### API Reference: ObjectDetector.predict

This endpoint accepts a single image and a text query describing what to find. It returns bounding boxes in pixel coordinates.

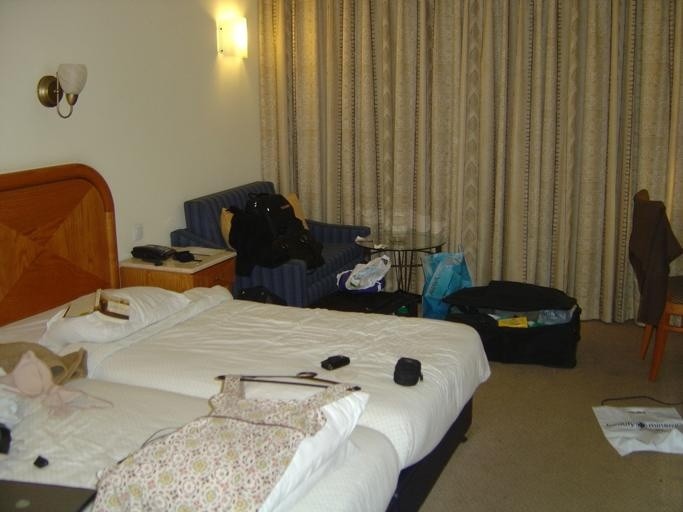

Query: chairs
[171,181,371,308]
[633,189,683,383]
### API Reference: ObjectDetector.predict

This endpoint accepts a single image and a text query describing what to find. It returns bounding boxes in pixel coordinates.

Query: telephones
[131,244,177,260]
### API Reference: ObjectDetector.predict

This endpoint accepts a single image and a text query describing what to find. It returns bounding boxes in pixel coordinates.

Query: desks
[354,229,446,312]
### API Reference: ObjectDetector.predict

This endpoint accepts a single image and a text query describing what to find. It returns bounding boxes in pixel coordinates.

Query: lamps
[217,5,250,62]
[38,64,85,118]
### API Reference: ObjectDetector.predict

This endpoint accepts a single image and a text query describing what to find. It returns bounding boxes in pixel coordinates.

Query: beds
[0,381,398,512]
[0,162,491,503]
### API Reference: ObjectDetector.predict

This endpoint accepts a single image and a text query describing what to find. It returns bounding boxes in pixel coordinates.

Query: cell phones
[321,356,350,369]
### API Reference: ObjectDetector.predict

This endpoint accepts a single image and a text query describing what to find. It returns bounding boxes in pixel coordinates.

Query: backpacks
[246,191,305,266]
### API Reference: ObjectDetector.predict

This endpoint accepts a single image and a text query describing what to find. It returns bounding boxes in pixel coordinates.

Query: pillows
[220,193,310,249]
[47,286,187,335]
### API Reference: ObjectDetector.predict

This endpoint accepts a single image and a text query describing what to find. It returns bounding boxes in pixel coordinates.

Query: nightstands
[120,249,238,300]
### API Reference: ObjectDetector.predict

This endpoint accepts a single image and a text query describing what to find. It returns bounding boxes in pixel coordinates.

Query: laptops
[0,479,96,512]
